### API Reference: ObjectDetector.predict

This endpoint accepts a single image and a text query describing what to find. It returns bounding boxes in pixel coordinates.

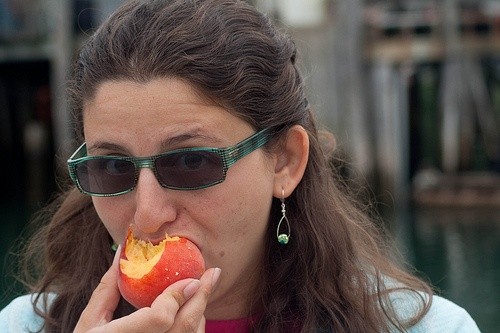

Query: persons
[0,0,482,333]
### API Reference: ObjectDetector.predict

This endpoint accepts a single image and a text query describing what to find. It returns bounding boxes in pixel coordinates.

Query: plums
[118,228,205,310]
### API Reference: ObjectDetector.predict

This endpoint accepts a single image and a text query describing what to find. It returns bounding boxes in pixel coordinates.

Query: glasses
[67,127,277,198]
